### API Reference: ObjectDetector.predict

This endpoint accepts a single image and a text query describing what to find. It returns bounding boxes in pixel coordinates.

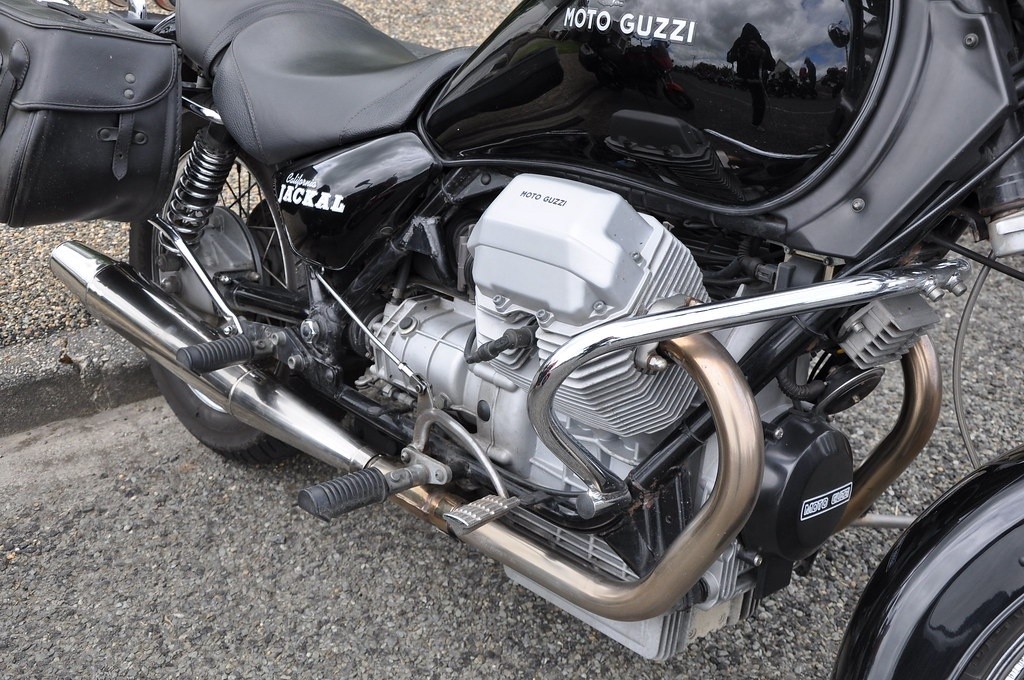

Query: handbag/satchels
[0,0,181,228]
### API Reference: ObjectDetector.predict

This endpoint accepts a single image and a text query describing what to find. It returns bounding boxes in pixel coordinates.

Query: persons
[726,23,816,132]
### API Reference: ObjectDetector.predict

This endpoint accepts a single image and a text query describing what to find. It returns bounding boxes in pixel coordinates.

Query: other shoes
[155,0,175,11]
[108,0,129,7]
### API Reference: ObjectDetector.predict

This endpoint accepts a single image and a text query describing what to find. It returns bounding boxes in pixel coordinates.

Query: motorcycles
[47,2,1024,679]
